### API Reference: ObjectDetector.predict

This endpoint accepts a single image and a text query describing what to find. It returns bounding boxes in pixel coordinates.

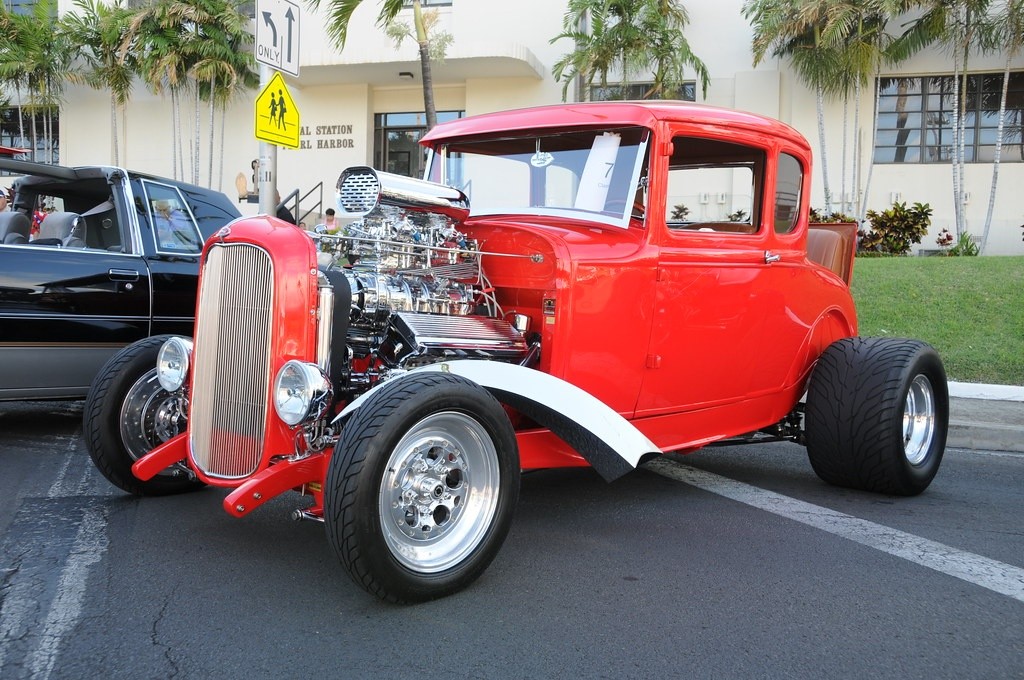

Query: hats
[0,186,10,196]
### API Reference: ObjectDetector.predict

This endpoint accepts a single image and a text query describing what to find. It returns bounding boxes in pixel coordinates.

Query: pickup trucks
[0,156,245,405]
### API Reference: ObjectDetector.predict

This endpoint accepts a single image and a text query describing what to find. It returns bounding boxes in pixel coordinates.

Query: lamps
[399,72,414,79]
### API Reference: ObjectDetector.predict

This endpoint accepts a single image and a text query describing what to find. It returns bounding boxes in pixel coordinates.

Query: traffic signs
[253,0,301,79]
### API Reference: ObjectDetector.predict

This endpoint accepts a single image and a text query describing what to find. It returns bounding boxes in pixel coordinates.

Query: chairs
[37,212,89,248]
[806,229,844,278]
[677,221,755,234]
[0,212,31,244]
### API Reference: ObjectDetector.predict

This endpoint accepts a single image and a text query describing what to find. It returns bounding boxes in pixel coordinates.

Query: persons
[154,199,195,245]
[276,188,343,230]
[0,190,11,212]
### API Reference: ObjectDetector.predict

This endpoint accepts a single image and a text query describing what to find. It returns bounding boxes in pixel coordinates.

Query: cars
[79,97,953,608]
